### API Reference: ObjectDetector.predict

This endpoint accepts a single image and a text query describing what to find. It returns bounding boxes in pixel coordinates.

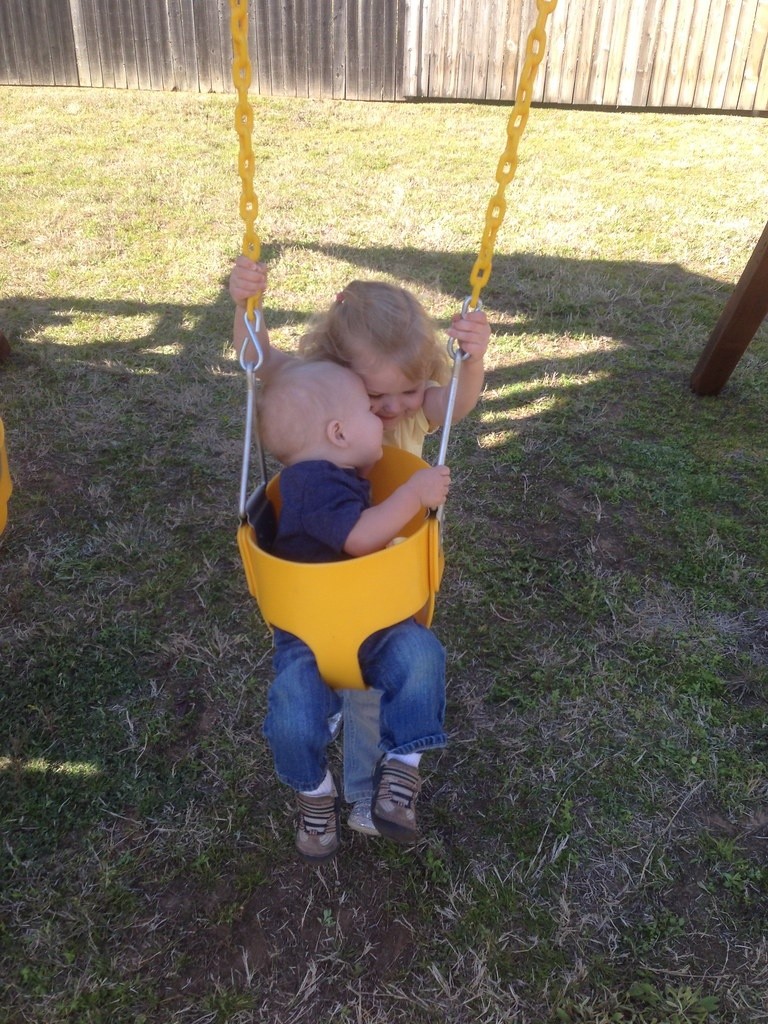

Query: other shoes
[346,813,379,835]
[326,710,342,743]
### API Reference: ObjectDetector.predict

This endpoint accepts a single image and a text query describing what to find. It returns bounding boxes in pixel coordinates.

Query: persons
[228,253,492,838]
[259,357,452,860]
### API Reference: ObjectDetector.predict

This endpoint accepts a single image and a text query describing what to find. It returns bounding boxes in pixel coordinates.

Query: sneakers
[372,757,422,831]
[294,790,338,857]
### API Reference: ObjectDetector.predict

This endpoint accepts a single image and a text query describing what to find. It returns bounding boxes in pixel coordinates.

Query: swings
[227,1,558,694]
